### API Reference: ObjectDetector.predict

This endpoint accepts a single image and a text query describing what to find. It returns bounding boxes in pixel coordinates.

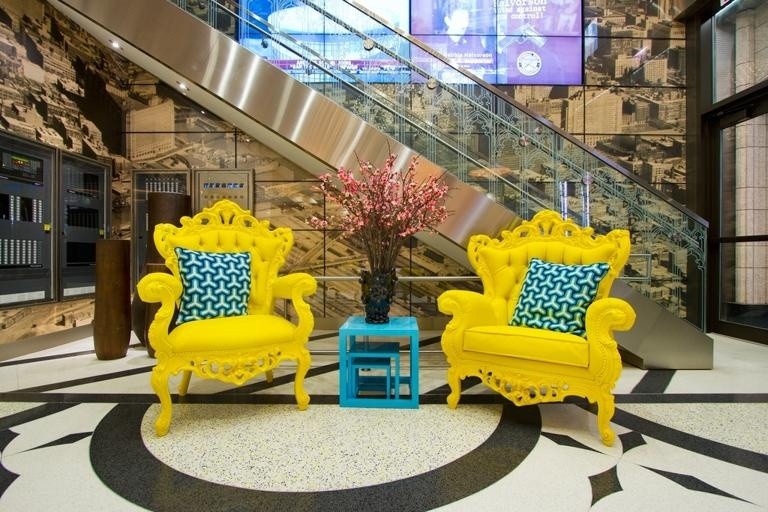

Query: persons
[433,1,495,85]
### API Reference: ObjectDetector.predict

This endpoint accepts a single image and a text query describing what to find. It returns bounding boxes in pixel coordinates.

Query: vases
[361,269,398,324]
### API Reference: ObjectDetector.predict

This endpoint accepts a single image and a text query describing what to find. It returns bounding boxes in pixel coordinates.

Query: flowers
[299,140,457,273]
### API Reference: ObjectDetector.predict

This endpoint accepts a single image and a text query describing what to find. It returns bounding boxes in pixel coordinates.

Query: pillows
[174,247,252,326]
[510,256,610,339]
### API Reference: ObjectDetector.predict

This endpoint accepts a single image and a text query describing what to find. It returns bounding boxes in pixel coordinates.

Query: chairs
[437,210,631,447]
[137,200,319,435]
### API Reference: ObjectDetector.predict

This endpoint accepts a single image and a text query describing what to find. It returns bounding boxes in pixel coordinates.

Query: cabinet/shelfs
[59,148,113,302]
[0,131,58,312]
